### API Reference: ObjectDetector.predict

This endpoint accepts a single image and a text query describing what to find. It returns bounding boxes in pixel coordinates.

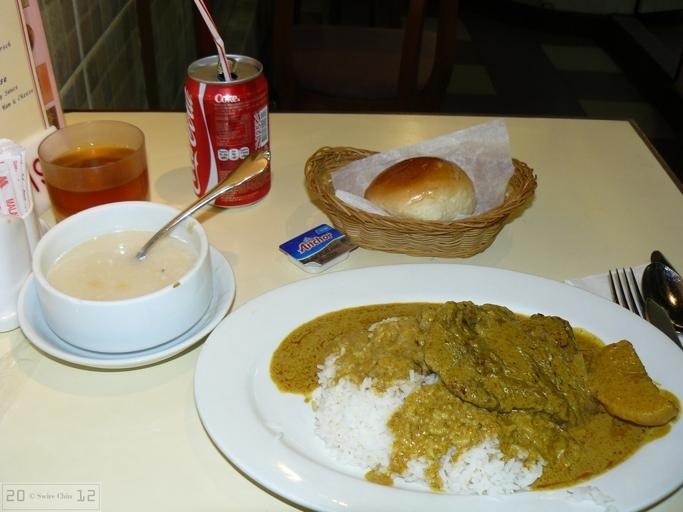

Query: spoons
[641,263,683,329]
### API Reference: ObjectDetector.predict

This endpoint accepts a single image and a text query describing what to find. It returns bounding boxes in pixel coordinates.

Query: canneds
[183,54,273,208]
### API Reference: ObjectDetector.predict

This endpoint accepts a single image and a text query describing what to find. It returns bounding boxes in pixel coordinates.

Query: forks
[608,267,646,320]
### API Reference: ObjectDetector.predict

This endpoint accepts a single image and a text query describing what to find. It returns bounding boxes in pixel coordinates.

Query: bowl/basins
[32,197,213,355]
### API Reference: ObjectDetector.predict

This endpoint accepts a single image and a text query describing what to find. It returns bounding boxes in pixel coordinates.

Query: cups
[37,120,149,223]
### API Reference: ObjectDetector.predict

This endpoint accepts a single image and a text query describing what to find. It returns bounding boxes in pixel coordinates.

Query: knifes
[651,251,681,283]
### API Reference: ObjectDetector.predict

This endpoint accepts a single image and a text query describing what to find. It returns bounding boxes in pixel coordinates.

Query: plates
[17,236,236,371]
[195,265,683,512]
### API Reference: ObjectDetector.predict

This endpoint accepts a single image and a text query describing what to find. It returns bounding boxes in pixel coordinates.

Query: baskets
[305,147,538,258]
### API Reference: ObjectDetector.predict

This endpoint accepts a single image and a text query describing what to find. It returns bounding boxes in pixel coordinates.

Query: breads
[362,157,478,223]
